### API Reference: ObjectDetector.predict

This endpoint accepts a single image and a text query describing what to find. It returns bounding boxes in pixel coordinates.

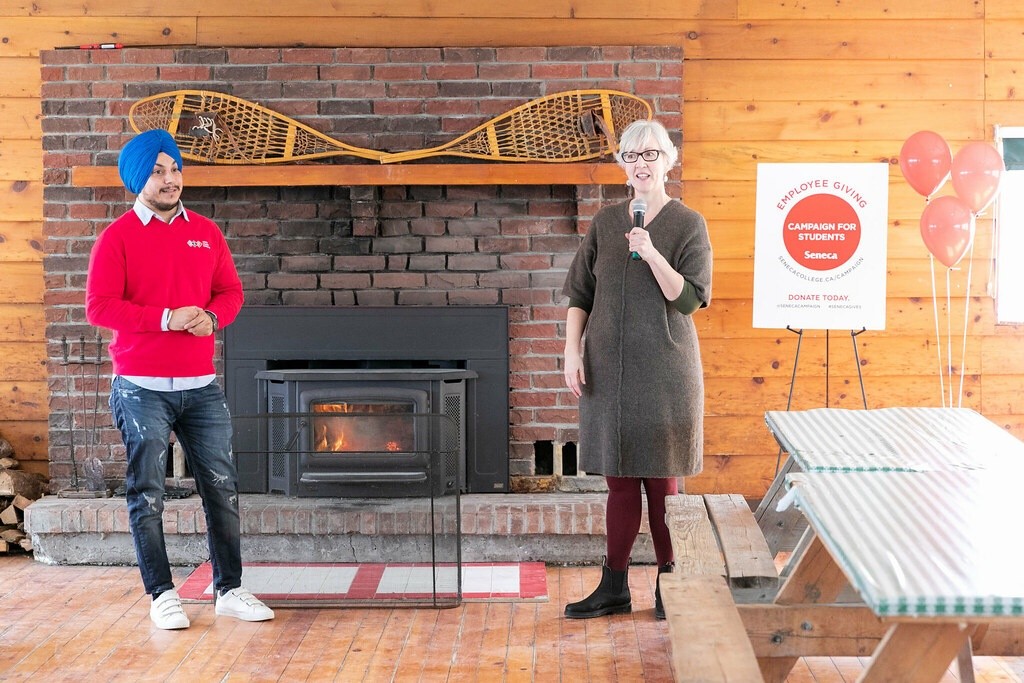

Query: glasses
[621,148,663,164]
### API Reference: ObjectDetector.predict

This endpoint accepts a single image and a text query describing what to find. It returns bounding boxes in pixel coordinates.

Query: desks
[783,467,1023,625]
[764,404,1023,472]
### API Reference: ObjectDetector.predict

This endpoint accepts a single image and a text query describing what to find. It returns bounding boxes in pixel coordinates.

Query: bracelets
[165,308,174,326]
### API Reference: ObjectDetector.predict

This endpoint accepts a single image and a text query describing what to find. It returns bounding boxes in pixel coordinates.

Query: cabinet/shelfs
[68,164,631,188]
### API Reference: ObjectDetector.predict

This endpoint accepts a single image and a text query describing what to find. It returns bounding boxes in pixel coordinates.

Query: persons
[562,120,714,619]
[84,131,277,630]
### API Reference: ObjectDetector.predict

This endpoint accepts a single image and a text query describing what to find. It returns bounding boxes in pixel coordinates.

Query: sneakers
[213,588,275,624]
[149,590,191,632]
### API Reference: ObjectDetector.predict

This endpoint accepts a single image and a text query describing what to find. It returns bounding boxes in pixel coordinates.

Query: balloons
[899,130,953,198]
[920,195,976,269]
[951,143,1005,218]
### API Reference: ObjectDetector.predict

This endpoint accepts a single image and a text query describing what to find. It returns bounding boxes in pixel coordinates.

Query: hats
[117,129,182,196]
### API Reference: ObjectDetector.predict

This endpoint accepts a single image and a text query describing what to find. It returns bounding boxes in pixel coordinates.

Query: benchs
[663,491,781,588]
[658,572,768,683]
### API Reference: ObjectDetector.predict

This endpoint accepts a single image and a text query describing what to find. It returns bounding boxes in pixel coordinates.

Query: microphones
[631,198,648,261]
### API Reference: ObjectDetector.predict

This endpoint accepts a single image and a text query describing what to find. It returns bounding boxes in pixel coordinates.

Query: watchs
[205,311,218,333]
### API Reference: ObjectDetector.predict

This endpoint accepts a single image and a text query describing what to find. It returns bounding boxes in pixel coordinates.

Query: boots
[564,556,633,619]
[655,563,675,618]
